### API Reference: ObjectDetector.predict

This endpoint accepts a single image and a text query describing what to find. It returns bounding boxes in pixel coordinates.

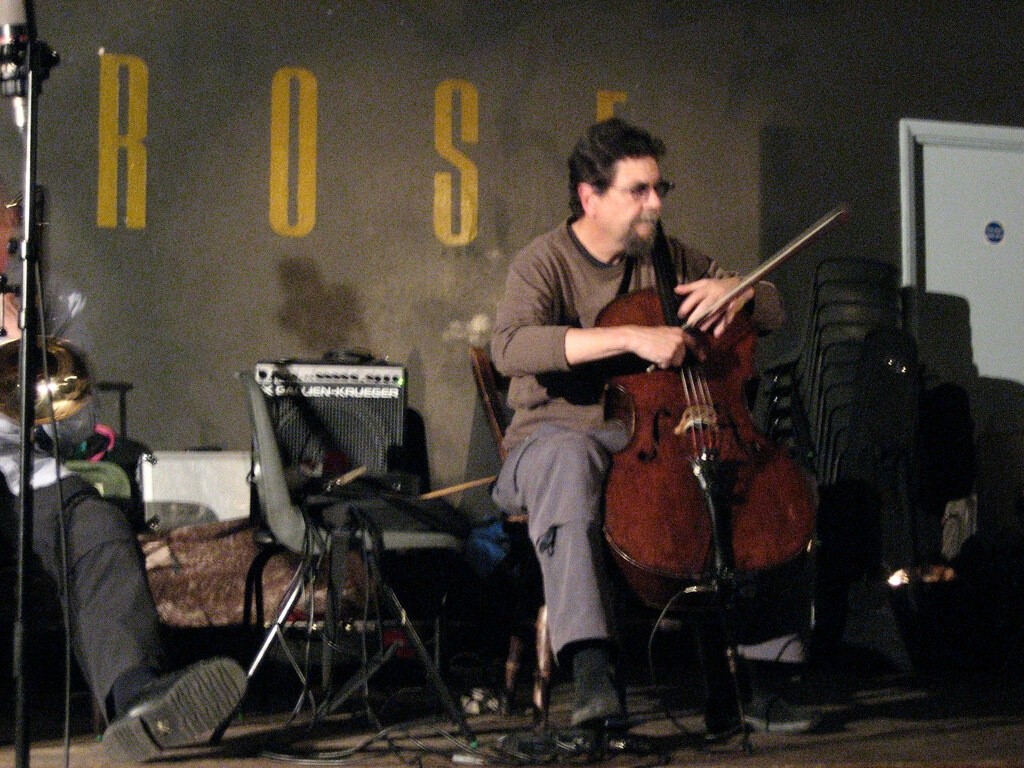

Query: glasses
[611,180,677,203]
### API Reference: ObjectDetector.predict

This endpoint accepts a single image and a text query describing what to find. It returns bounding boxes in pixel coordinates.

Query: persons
[490,120,815,735]
[0,194,247,764]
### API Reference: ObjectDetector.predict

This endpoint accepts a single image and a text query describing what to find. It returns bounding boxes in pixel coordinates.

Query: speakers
[248,361,407,534]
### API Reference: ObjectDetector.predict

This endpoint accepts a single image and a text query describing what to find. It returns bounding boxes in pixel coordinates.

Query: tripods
[211,502,482,752]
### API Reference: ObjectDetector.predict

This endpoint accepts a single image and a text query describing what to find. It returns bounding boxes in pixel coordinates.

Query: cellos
[572,174,832,761]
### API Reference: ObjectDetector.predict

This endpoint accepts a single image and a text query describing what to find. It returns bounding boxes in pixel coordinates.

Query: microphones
[0,0,32,132]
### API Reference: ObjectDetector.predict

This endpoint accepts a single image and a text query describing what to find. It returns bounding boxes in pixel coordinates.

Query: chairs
[469,346,727,731]
[765,255,930,647]
[242,372,462,711]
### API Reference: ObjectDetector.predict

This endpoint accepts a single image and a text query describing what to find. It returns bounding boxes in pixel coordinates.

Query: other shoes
[570,648,629,728]
[101,658,247,765]
[730,677,811,734]
